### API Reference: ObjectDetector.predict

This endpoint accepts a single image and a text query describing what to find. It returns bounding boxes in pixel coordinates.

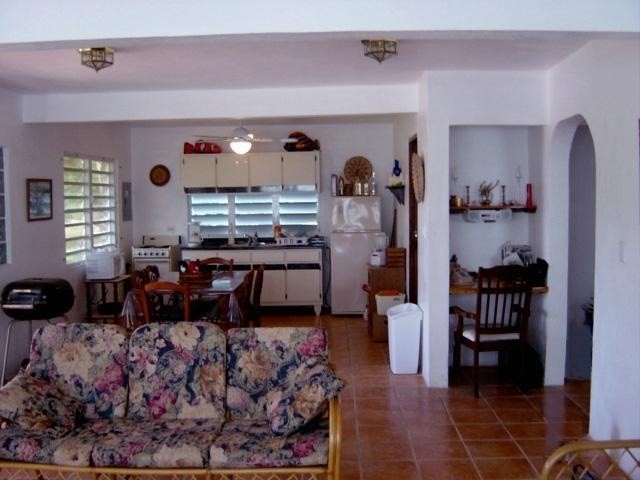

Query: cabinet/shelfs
[252,249,323,305]
[181,154,249,188]
[367,266,405,343]
[248,150,321,187]
[181,249,251,304]
[450,206,548,295]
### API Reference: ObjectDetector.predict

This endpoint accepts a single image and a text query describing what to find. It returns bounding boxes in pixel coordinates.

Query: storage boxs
[375,291,406,316]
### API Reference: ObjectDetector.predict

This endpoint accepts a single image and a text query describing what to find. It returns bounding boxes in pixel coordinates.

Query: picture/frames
[26,179,53,221]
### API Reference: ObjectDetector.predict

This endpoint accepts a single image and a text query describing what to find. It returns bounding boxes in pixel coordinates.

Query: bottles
[272,222,282,242]
[228,223,236,245]
[328,171,379,197]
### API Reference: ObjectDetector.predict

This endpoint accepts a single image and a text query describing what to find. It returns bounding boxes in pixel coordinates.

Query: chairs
[449,265,531,398]
[538,440,640,480]
[130,257,264,329]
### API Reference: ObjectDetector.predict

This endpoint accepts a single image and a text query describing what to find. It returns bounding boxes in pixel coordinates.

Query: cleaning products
[275,220,282,240]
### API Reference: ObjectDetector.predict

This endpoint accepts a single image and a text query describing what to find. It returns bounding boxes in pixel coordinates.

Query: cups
[186,221,205,249]
[188,259,201,273]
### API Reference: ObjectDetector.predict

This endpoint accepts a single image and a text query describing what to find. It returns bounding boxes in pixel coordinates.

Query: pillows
[0,368,83,438]
[265,355,344,438]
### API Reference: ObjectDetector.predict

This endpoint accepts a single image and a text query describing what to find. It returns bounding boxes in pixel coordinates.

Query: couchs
[0,321,341,480]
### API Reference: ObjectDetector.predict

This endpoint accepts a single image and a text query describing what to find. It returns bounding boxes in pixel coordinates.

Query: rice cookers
[369,247,385,268]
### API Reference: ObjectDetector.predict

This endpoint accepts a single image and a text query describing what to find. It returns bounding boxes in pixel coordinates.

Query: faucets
[243,234,257,243]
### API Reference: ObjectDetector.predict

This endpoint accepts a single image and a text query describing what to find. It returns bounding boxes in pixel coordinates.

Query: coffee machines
[328,197,389,317]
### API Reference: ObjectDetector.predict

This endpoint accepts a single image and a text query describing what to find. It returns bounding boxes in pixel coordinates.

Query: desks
[1,315,71,388]
[81,274,130,324]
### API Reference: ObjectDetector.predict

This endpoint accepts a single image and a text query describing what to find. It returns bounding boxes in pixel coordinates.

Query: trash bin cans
[387,302,422,375]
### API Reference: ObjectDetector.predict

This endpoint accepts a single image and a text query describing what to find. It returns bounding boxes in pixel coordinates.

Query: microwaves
[83,252,128,280]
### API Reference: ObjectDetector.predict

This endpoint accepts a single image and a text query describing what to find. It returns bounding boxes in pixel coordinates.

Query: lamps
[79,47,115,72]
[361,39,400,63]
[230,142,251,154]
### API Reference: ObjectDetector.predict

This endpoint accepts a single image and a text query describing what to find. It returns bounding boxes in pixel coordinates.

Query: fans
[192,121,297,145]
